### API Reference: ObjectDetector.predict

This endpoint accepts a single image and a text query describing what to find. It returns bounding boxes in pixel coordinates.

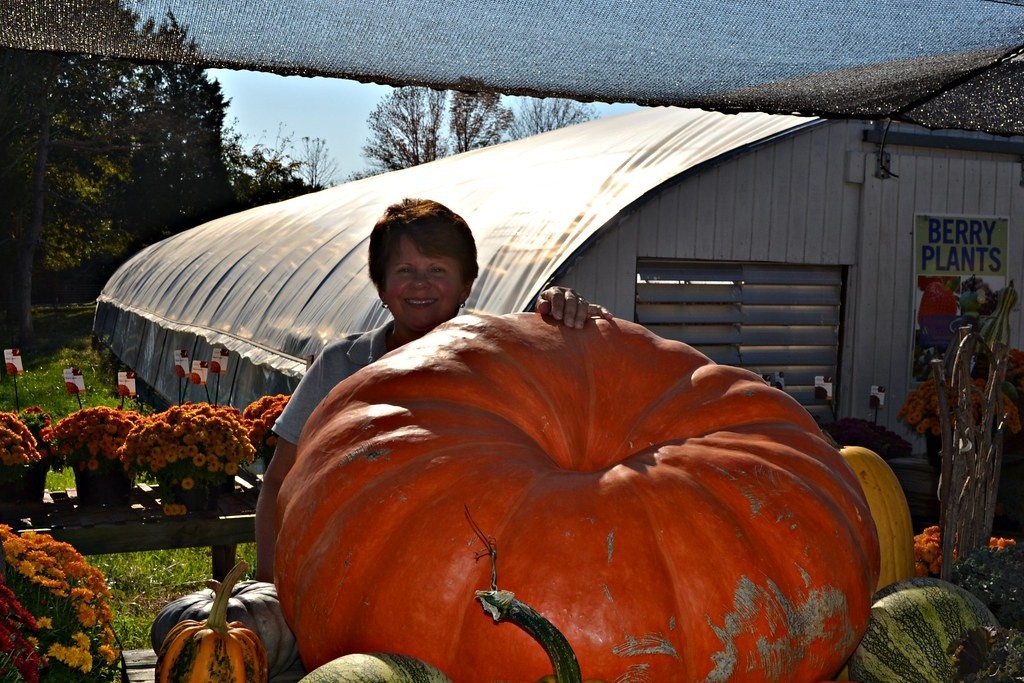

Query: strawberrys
[918,276,961,328]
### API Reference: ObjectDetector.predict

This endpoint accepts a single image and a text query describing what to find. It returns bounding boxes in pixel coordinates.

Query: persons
[250,197,617,586]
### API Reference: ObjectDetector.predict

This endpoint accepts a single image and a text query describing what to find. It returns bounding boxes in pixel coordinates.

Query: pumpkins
[973,278,1020,354]
[272,308,879,682]
[820,429,914,593]
[295,651,455,682]
[849,577,1004,682]
[149,561,304,683]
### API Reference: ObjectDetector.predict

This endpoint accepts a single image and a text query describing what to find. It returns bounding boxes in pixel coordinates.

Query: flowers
[49,405,146,473]
[241,393,292,459]
[0,405,54,483]
[116,400,257,516]
[0,523,123,683]
[910,524,1017,579]
[895,373,1022,435]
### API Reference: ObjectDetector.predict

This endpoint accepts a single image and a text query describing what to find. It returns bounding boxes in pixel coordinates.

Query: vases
[154,457,236,510]
[924,429,956,469]
[0,460,49,503]
[70,460,135,510]
